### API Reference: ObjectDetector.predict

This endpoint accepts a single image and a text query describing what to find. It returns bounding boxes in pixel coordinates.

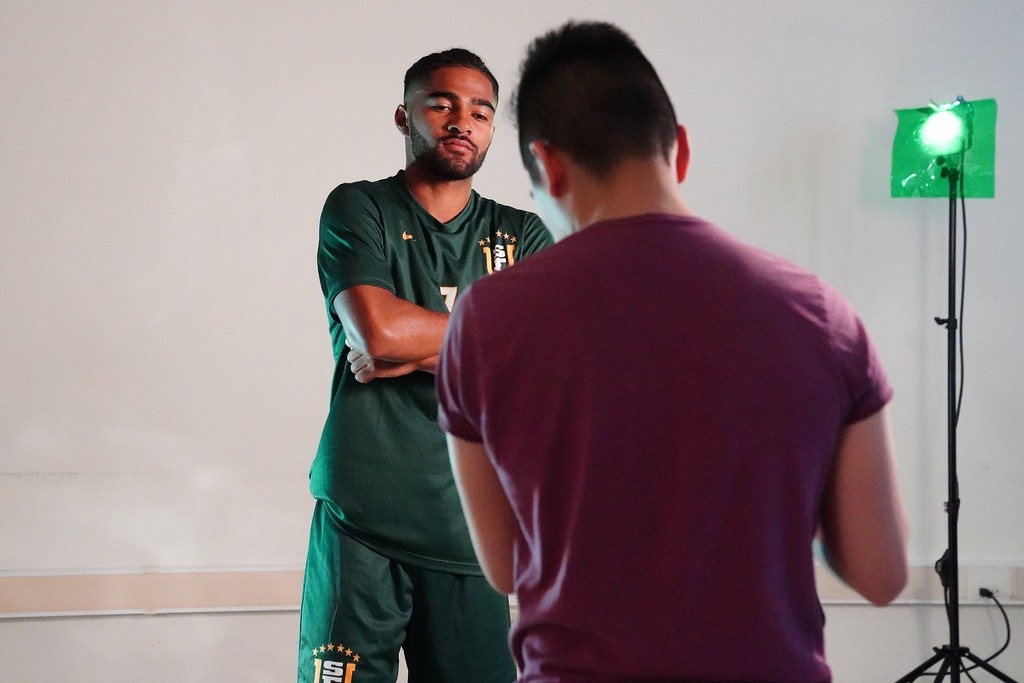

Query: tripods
[898,172,1013,683]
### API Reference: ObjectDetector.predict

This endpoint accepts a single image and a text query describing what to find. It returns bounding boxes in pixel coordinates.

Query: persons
[296,47,554,683]
[436,19,906,683]
